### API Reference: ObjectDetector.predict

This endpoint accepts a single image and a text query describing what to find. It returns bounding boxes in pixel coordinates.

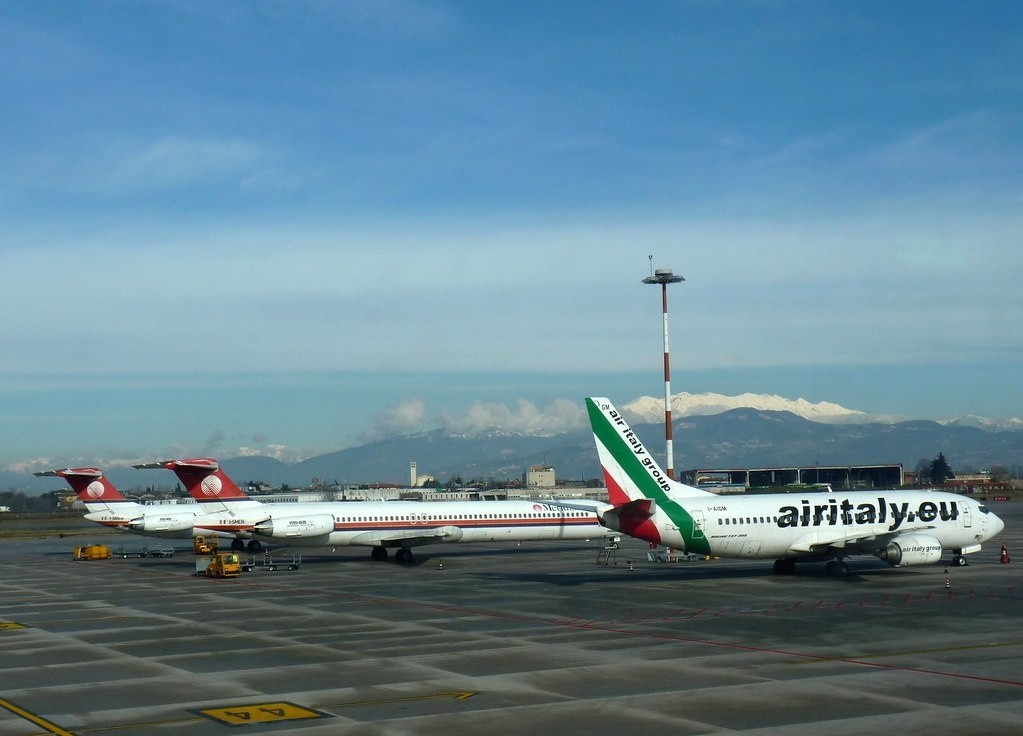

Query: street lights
[640,253,686,563]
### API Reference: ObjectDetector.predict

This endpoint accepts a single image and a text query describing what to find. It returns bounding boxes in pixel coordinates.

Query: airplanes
[32,456,626,564]
[529,396,1005,577]
